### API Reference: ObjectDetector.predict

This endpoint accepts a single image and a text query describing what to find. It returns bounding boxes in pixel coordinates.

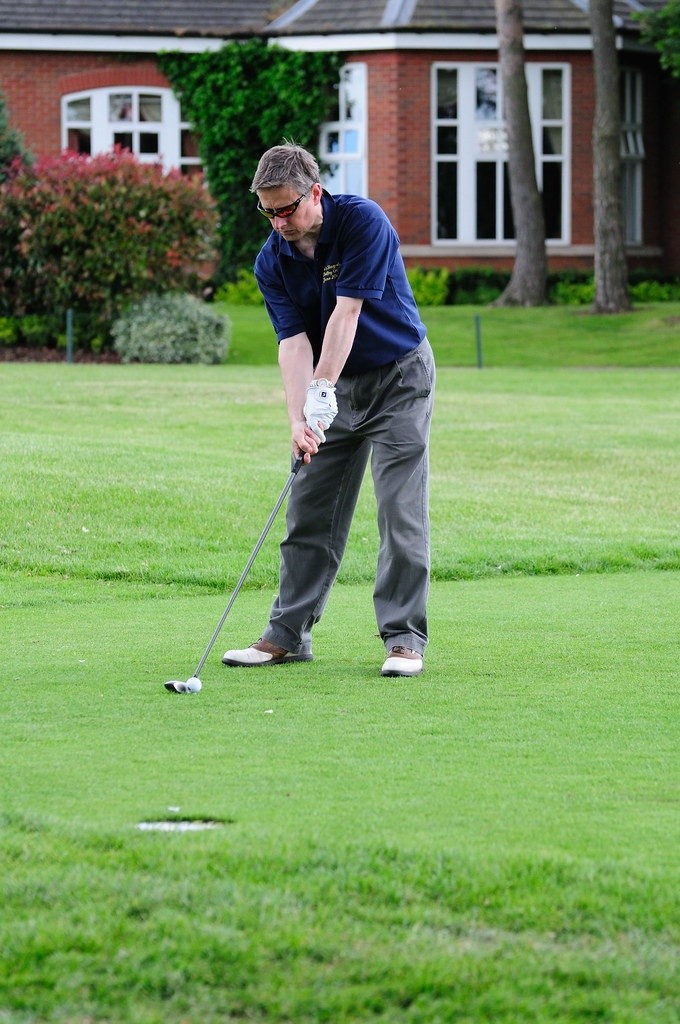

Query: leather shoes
[222,639,313,667]
[382,646,423,676]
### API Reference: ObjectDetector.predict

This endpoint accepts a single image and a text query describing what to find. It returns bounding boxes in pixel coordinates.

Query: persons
[219,136,433,677]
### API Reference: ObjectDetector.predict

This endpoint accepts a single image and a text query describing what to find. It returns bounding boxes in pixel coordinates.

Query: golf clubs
[163,427,312,695]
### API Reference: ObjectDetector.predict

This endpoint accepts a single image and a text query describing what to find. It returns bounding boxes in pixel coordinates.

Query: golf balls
[184,677,202,694]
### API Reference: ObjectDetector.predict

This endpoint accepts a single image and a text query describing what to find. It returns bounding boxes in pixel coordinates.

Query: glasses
[257,195,305,219]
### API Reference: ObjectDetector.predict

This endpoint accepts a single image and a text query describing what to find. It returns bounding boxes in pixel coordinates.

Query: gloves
[303,386,338,444]
